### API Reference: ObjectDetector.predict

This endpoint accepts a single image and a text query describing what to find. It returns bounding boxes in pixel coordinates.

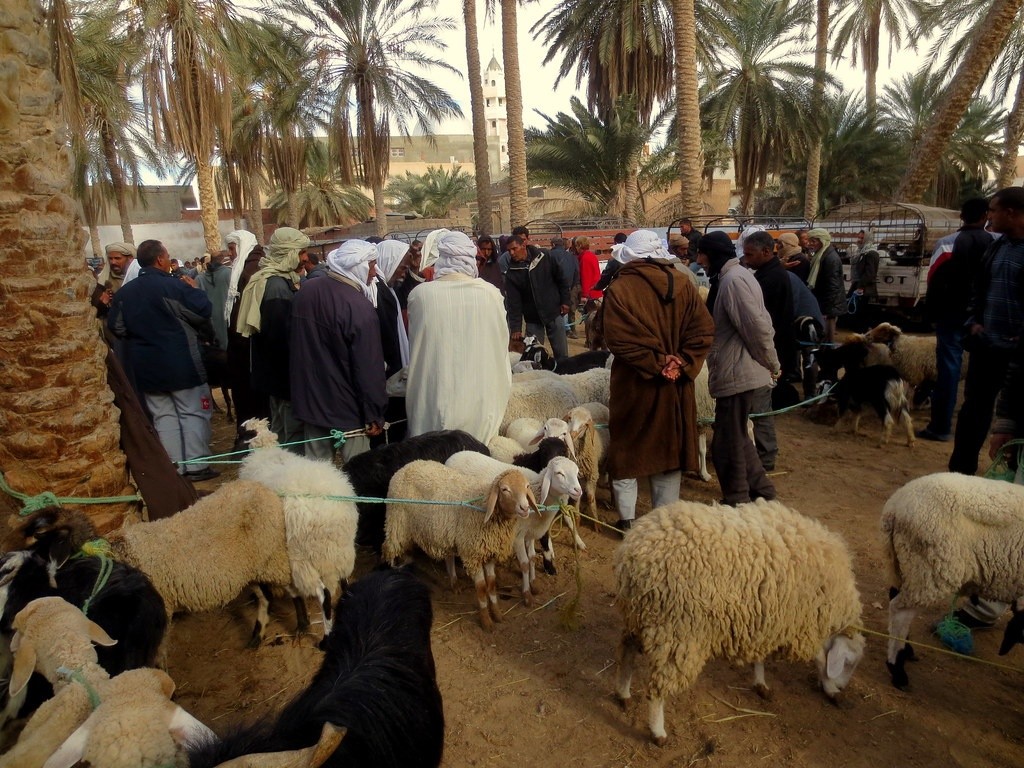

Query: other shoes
[917,429,950,443]
[187,466,220,481]
[933,605,994,634]
[616,517,634,541]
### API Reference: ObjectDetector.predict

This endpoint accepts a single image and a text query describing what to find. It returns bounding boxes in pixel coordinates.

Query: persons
[695,233,784,507]
[91,226,631,548]
[602,231,717,539]
[668,187,1024,476]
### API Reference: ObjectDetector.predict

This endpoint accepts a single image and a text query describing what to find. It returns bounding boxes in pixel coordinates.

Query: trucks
[382,225,500,264]
[810,199,1004,332]
[666,214,810,245]
[523,215,639,273]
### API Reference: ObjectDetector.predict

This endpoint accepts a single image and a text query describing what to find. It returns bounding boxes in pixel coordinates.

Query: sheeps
[506,417,587,552]
[446,450,583,608]
[880,471,1024,692]
[239,419,357,649]
[614,499,866,746]
[520,342,612,374]
[498,379,582,441]
[384,337,533,398]
[509,360,612,408]
[690,362,756,482]
[0,505,446,768]
[797,315,970,447]
[563,402,611,437]
[573,427,608,534]
[486,434,572,577]
[92,476,312,651]
[380,459,542,632]
